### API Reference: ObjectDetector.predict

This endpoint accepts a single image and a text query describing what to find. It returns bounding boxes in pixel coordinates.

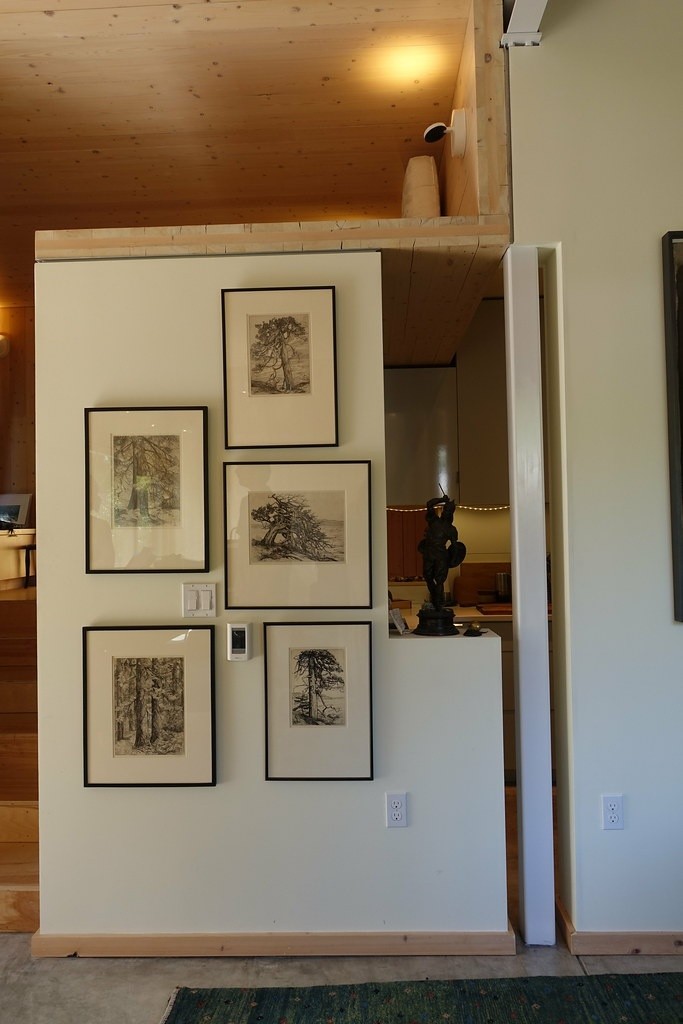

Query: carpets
[159,972,683,1024]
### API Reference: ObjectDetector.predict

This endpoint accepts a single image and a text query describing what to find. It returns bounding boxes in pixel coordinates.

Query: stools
[22,543,36,589]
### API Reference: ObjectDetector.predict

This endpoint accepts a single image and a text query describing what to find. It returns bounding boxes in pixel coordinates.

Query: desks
[452,606,552,769]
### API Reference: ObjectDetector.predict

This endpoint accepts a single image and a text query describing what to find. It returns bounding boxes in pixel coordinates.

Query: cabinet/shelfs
[385,300,549,507]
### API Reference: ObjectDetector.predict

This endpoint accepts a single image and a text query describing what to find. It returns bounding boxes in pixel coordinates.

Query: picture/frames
[84,406,210,573]
[223,460,372,609]
[81,625,216,788]
[262,622,373,781]
[221,285,339,449]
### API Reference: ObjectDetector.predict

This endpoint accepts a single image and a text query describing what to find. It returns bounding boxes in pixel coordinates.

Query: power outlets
[602,795,624,830]
[386,792,407,827]
